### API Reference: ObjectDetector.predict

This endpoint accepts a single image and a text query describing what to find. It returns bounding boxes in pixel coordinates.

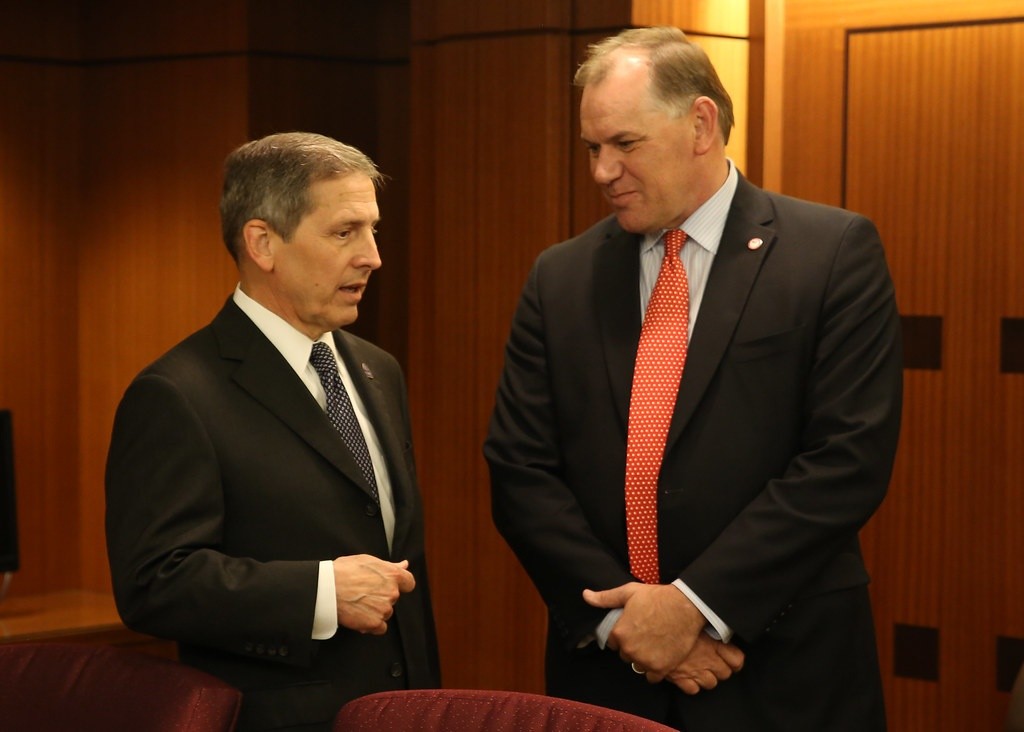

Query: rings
[632,663,645,674]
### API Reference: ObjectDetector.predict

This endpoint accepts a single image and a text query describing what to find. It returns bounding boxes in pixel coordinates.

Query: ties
[310,343,381,509]
[624,232,691,584]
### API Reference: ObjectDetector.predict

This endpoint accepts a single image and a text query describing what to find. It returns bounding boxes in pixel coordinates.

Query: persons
[104,134,442,732]
[483,27,903,732]
